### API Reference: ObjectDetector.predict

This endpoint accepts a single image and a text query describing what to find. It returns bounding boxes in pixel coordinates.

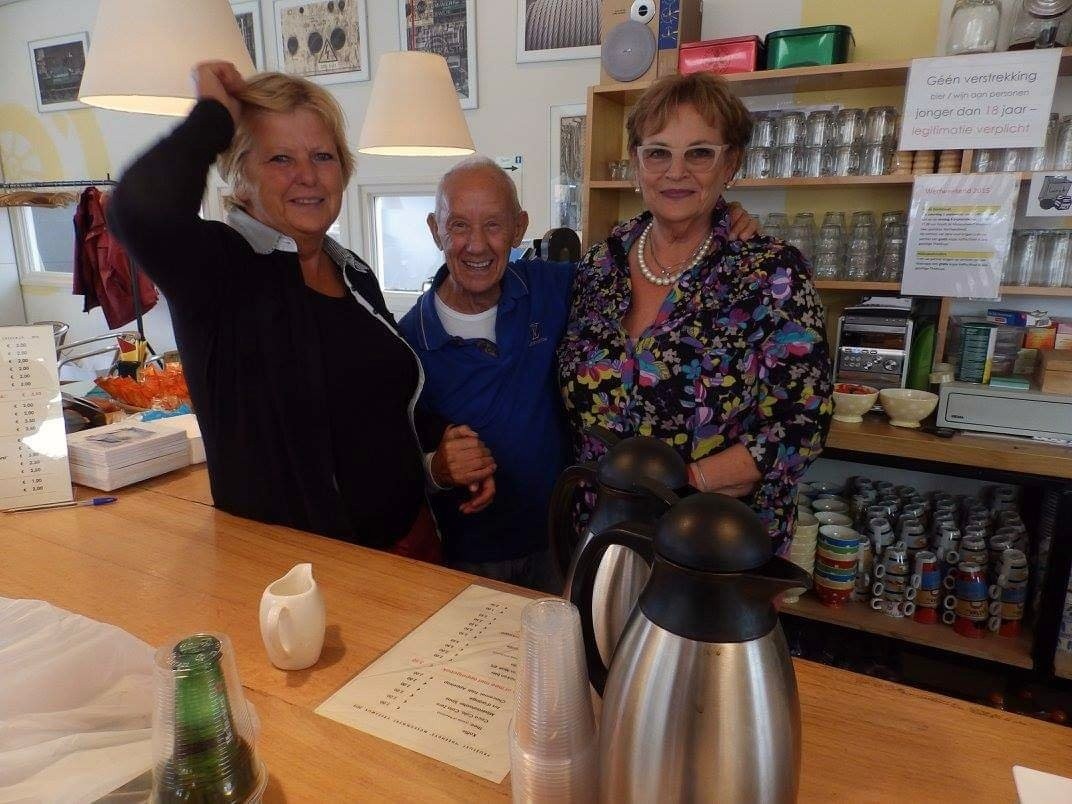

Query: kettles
[568,492,816,804]
[544,436,691,694]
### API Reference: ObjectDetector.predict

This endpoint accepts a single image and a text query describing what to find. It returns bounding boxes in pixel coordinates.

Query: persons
[105,60,495,567]
[553,71,836,613]
[400,157,762,594]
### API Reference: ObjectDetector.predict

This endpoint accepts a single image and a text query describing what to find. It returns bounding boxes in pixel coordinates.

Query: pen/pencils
[0,498,117,513]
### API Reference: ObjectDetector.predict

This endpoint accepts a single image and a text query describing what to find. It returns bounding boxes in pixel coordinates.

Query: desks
[0,461,1069,804]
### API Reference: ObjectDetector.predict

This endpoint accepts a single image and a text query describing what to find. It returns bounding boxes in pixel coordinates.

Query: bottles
[150,635,264,804]
[1008,0,1071,51]
[749,211,815,282]
[944,1,1003,56]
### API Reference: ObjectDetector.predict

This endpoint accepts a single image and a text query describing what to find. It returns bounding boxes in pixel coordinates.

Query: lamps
[357,0,477,156]
[76,0,255,117]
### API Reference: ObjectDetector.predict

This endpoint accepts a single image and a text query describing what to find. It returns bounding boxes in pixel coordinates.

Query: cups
[779,478,861,609]
[805,106,898,176]
[996,148,1025,172]
[1030,113,1072,171]
[147,630,270,804]
[850,476,940,625]
[972,148,999,173]
[816,210,878,282]
[609,159,630,182]
[932,487,1029,641]
[1002,229,1071,287]
[879,210,908,282]
[733,113,805,178]
[507,597,600,804]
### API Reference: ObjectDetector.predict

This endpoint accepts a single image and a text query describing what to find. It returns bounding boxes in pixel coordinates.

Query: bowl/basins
[879,389,939,428]
[831,384,879,423]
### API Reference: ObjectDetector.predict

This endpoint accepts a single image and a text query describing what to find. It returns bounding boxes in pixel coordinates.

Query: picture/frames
[273,0,370,85]
[28,32,95,113]
[399,0,478,110]
[231,0,264,74]
[516,0,601,64]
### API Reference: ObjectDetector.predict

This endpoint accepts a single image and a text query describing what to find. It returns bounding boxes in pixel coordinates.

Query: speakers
[599,0,660,85]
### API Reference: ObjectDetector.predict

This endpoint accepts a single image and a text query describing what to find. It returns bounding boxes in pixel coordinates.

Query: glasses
[637,145,729,174]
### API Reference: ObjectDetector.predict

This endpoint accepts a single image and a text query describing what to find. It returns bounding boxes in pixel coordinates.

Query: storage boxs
[765,25,851,70]
[679,35,764,77]
[987,308,1072,397]
[601,0,702,85]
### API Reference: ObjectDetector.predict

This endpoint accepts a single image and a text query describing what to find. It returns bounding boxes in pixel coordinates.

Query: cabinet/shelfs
[581,45,1072,727]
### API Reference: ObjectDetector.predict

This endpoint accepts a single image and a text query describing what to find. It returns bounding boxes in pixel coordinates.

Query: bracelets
[687,461,710,492]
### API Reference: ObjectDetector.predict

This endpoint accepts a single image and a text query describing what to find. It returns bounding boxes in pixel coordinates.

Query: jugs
[259,562,324,670]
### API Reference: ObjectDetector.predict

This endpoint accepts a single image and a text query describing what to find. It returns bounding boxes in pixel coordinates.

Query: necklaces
[638,221,715,286]
